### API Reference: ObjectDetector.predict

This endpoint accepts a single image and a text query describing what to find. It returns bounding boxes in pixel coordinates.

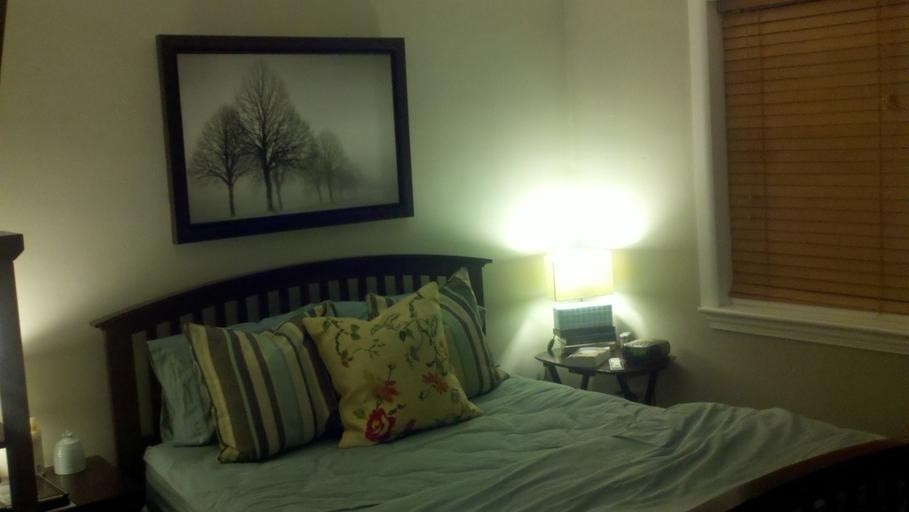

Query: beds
[91,254,908,511]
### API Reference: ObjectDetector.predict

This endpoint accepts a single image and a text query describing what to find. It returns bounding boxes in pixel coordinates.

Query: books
[551,324,619,369]
[0,473,69,511]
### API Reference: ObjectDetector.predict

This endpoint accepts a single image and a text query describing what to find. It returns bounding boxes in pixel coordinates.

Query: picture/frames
[157,36,413,244]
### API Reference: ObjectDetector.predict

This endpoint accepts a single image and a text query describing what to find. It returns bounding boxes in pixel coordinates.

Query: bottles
[53,430,87,475]
[28,415,46,476]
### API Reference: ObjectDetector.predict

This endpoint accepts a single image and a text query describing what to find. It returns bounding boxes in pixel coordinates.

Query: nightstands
[44,456,145,511]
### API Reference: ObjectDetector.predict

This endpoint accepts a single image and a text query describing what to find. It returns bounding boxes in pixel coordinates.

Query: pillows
[303,282,482,450]
[184,300,343,464]
[146,303,341,446]
[339,295,411,322]
[367,268,510,400]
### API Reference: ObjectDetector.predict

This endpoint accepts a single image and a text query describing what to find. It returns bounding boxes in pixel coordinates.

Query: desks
[535,346,669,405]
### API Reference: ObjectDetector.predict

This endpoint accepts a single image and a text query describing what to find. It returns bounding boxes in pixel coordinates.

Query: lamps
[553,253,616,346]
